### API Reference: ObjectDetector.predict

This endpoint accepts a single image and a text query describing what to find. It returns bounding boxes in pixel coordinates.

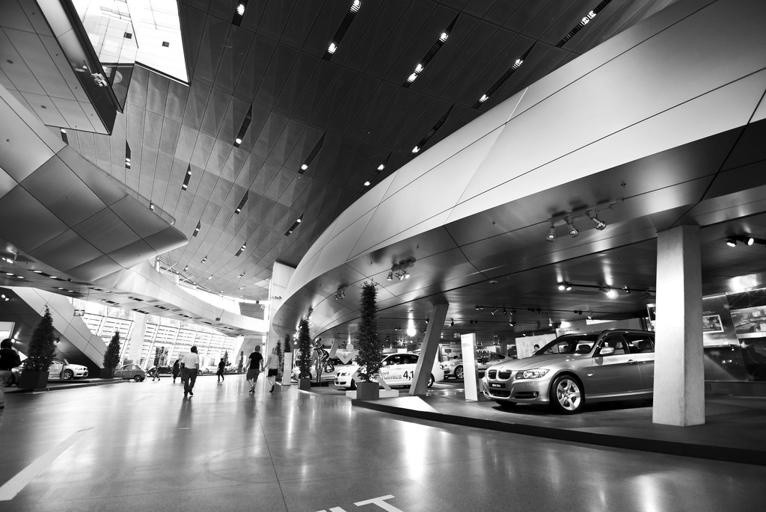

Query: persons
[170,360,178,383]
[151,363,161,382]
[179,363,185,384]
[261,346,279,393]
[244,345,264,394]
[181,345,199,398]
[216,358,225,383]
[0,338,22,411]
[532,344,540,354]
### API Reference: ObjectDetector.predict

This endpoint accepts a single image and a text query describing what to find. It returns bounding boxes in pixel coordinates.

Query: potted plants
[351,282,386,402]
[295,316,313,392]
[100,330,122,379]
[17,301,59,394]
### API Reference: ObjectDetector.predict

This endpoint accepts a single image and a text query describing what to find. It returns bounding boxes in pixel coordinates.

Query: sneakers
[184,389,194,398]
[269,385,275,393]
[249,385,256,394]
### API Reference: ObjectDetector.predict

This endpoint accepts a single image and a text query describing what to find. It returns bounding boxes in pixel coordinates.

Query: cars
[11,356,88,381]
[482,326,655,414]
[288,352,516,391]
[110,364,245,382]
